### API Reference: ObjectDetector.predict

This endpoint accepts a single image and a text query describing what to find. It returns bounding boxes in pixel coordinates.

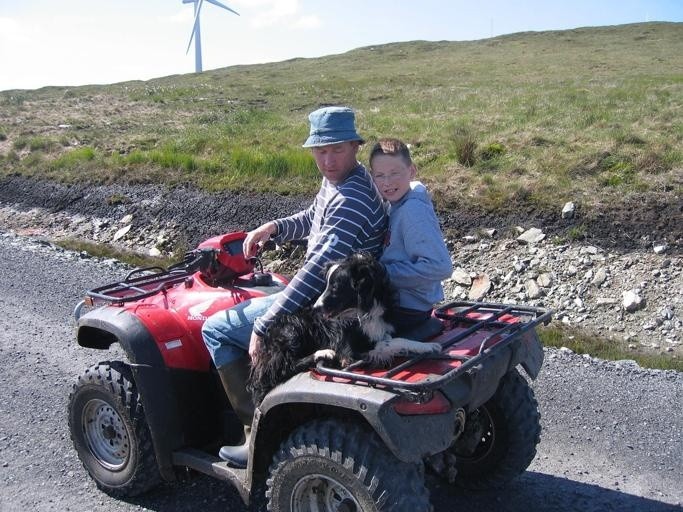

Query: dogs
[245,253,443,408]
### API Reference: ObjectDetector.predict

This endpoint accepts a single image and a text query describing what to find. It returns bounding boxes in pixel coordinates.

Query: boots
[217,352,254,468]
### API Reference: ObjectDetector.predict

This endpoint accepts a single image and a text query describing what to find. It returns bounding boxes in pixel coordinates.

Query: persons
[201,107,387,468]
[370,138,452,327]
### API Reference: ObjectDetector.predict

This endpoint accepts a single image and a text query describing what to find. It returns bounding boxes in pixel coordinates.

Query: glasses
[375,170,401,181]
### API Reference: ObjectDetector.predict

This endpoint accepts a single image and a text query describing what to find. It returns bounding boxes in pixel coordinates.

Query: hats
[302,107,365,148]
